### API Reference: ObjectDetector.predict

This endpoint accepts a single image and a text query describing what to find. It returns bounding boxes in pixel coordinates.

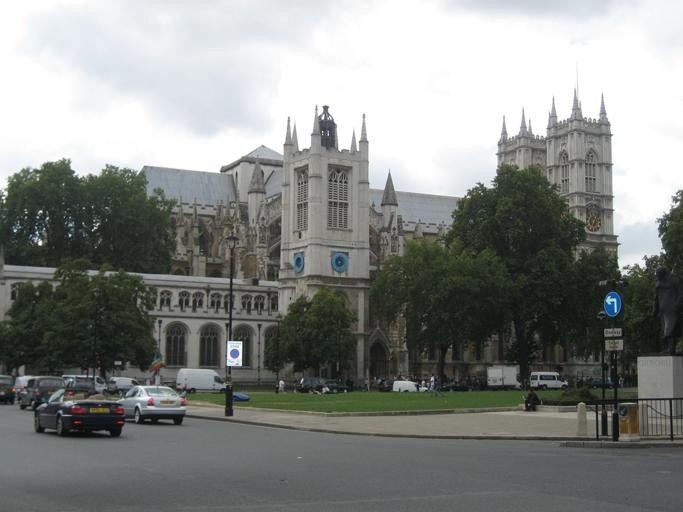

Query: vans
[529,371,569,391]
[389,379,432,393]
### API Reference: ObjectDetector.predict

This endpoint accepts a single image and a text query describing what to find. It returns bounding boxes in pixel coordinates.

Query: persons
[574,370,636,388]
[274,373,486,393]
[650,267,681,356]
[523,388,541,411]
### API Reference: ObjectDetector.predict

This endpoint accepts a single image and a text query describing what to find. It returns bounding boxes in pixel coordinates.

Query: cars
[591,379,617,390]
[0,369,189,439]
[295,376,350,394]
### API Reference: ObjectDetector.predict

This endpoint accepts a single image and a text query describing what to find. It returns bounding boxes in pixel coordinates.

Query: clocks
[586,211,601,232]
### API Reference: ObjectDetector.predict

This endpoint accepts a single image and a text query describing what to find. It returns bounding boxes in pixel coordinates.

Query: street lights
[256,322,262,387]
[598,278,630,442]
[154,319,163,386]
[223,231,241,418]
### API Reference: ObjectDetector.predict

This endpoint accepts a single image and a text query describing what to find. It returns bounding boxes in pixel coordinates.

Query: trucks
[174,368,229,393]
[486,365,525,390]
